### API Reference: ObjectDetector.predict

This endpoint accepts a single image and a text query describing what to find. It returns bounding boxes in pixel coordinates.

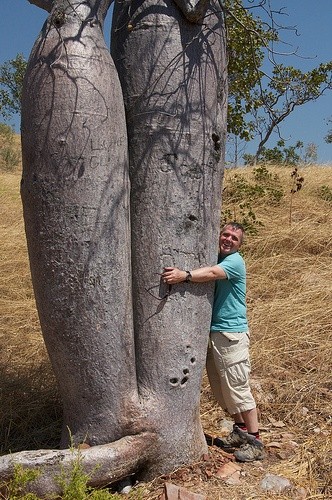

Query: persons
[163,220,268,464]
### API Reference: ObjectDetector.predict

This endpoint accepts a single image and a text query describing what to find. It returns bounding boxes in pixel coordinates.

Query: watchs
[181,270,192,285]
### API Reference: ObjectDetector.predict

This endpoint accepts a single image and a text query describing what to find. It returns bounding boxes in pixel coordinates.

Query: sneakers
[214,423,248,450]
[234,433,264,462]
